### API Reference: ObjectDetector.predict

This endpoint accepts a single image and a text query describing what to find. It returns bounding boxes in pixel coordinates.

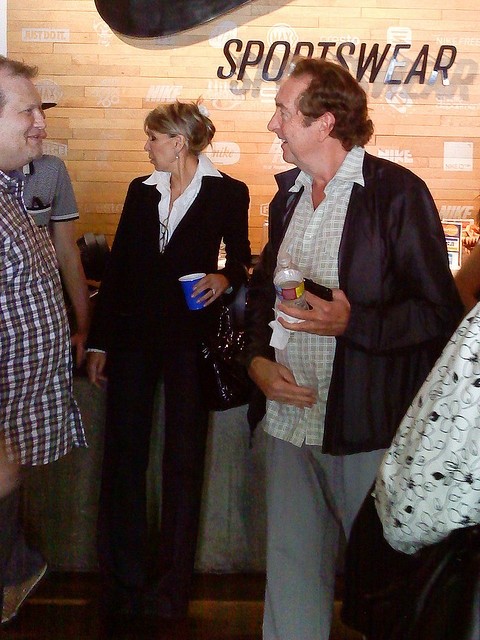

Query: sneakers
[1,562,49,625]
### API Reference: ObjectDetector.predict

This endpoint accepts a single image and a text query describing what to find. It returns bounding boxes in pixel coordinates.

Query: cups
[178,271,211,312]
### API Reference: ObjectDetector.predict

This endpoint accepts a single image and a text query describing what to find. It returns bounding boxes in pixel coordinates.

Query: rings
[210,289,215,295]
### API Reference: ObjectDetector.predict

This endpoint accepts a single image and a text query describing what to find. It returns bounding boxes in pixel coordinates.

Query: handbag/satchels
[195,259,250,412]
[341,482,479,640]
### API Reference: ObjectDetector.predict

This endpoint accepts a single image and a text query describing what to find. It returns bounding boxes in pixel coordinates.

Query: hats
[41,102,57,110]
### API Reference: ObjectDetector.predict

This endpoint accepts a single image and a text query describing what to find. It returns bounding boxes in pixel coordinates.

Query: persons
[453,236,480,314]
[0,53,88,623]
[14,103,92,368]
[243,59,465,640]
[84,102,252,622]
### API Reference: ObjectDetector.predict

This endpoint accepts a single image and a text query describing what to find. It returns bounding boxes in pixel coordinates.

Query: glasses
[156,217,169,254]
[32,195,43,208]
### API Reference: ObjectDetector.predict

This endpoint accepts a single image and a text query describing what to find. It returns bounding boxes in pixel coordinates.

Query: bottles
[272,252,309,334]
